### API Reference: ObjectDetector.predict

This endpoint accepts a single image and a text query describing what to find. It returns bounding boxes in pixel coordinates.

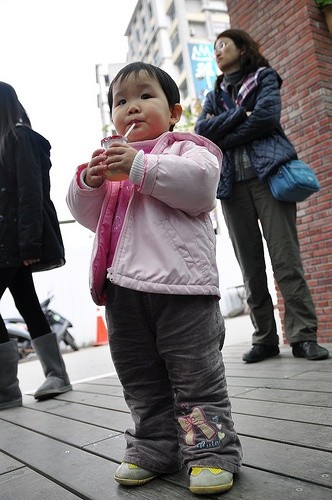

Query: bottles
[99,135,130,182]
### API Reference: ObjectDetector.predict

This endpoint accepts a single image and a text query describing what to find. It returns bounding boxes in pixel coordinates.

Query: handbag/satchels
[267,159,321,202]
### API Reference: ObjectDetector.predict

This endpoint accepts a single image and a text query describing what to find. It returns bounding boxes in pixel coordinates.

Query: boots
[0,340,23,409]
[29,331,73,399]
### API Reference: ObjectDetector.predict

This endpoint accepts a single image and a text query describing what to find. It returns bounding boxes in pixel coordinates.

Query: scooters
[2,290,80,360]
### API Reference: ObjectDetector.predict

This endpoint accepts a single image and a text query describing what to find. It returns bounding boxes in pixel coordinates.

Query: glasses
[213,40,236,56]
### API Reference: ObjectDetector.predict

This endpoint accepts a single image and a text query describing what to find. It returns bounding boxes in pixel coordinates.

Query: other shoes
[292,340,328,360]
[188,466,233,494]
[114,461,157,486]
[242,346,279,362]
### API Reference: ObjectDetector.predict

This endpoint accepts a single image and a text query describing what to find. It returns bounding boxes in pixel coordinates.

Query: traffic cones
[91,308,109,346]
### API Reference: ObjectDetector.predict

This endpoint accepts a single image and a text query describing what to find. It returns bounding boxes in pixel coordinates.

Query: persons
[0,80,73,411]
[65,62,243,495]
[195,29,329,362]
